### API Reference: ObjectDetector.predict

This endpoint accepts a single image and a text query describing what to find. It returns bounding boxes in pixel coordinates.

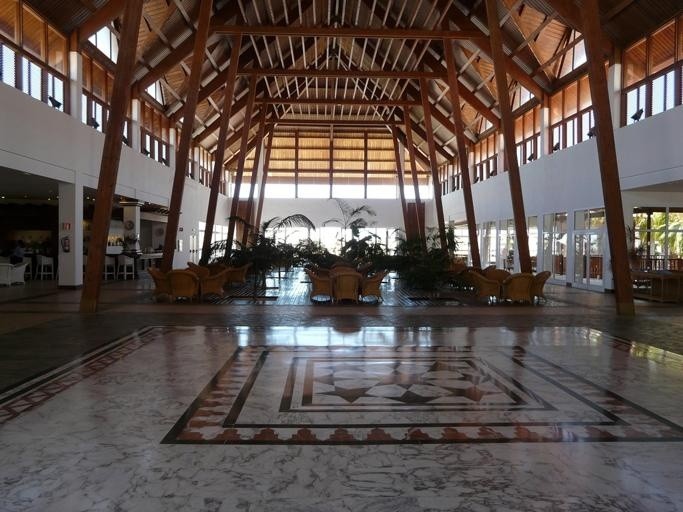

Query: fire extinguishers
[61,236,70,252]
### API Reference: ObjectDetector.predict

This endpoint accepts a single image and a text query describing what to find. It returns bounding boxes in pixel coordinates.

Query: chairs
[0,255,55,286]
[83,253,135,283]
[464,265,551,304]
[301,263,390,304]
[147,261,253,303]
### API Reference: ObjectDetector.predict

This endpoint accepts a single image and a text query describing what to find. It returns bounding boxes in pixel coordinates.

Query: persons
[15,240,26,263]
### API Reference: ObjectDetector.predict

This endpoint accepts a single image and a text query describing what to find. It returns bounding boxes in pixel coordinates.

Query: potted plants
[629,244,647,270]
